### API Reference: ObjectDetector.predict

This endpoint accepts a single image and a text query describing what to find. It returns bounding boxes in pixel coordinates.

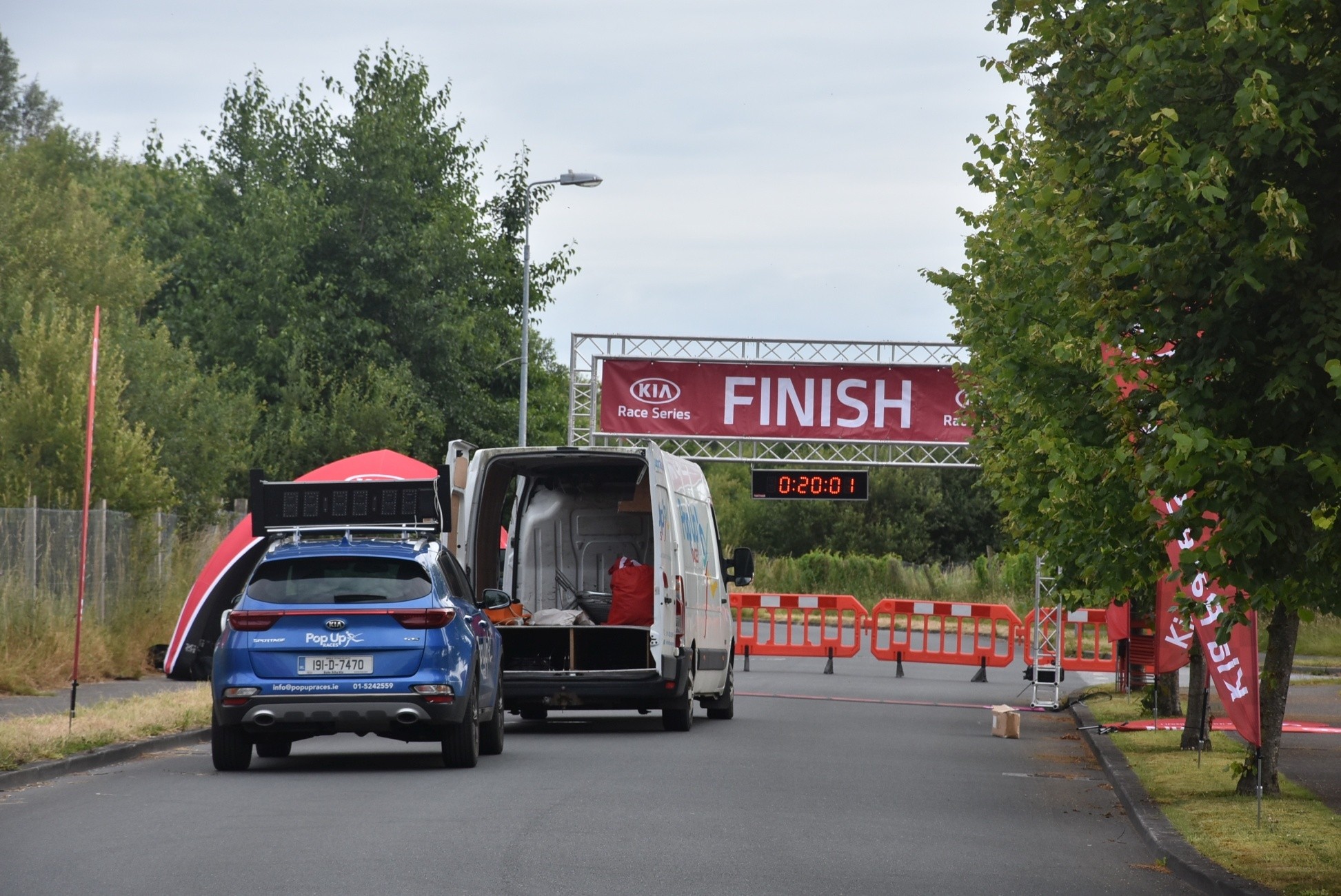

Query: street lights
[517,171,604,450]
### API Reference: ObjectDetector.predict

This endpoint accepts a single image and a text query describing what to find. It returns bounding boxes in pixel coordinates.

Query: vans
[442,438,757,734]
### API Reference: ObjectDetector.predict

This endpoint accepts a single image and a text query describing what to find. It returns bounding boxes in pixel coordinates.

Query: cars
[210,458,513,772]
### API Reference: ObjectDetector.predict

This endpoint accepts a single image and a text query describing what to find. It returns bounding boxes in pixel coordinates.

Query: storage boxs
[991,703,1020,739]
[485,603,522,626]
